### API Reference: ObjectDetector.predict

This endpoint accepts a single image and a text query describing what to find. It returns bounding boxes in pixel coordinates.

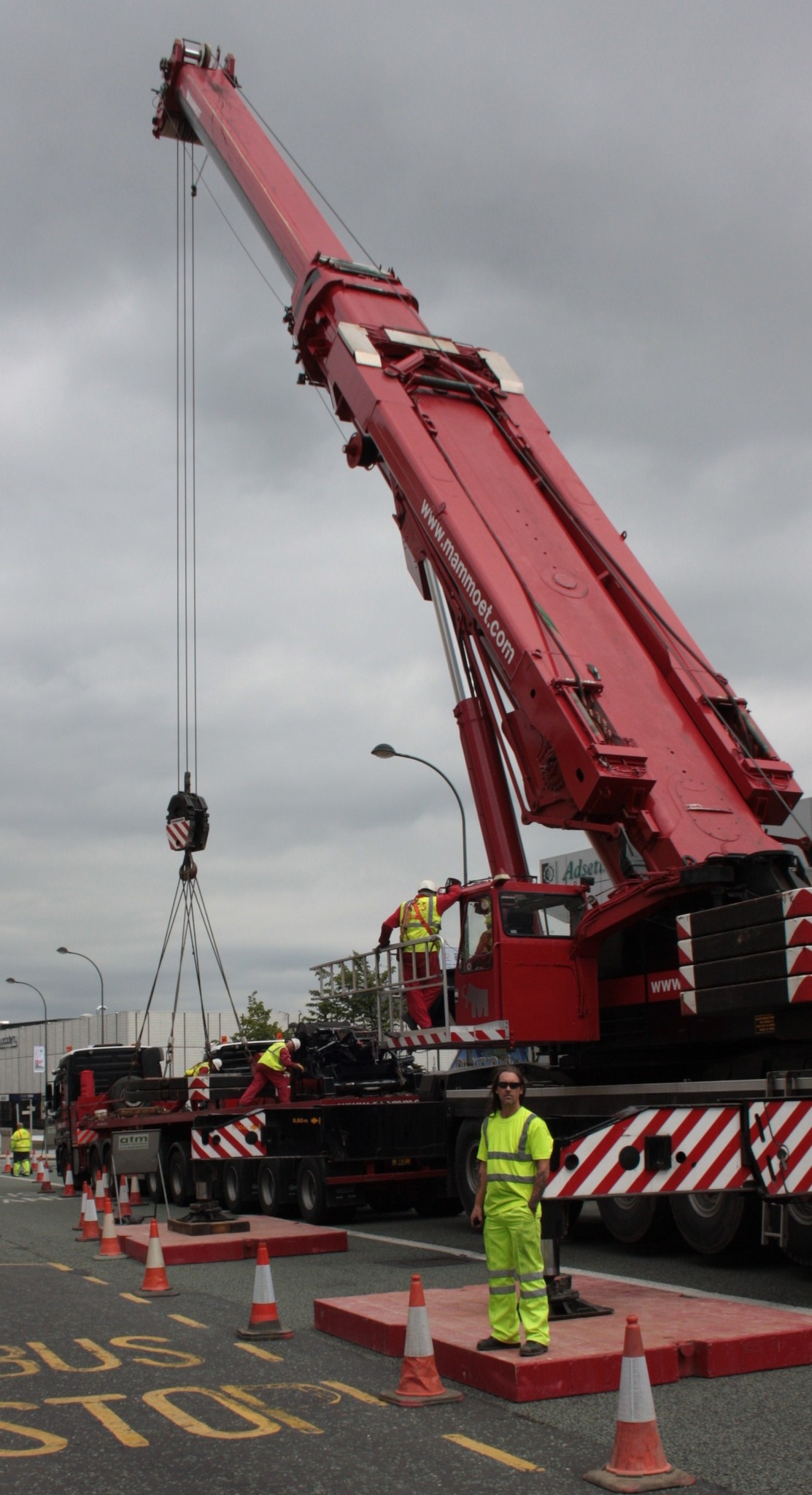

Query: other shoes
[401,1012,419,1031]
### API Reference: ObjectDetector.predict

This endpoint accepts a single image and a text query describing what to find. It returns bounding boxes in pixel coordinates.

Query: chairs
[505,912,535,935]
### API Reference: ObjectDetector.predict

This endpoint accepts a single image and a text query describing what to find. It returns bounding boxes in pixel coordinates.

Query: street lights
[55,945,109,1044]
[4,978,50,1151]
[368,744,472,965]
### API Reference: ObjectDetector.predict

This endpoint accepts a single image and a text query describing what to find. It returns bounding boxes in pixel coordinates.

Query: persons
[236,1038,305,1107]
[467,1066,555,1357]
[185,1058,222,1077]
[376,880,461,1031]
[10,1122,32,1177]
[471,910,495,970]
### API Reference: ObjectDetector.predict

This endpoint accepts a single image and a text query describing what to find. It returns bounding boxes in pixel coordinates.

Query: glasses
[497,1081,522,1089]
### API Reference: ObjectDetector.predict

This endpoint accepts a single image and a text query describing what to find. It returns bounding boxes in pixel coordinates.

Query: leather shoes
[519,1340,548,1357]
[476,1336,521,1351]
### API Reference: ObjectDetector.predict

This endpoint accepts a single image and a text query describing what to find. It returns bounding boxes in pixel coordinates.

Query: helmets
[291,1038,301,1052]
[212,1058,223,1071]
[418,880,437,893]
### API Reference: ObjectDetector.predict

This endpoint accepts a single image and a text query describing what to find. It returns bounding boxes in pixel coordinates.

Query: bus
[51,1041,165,1105]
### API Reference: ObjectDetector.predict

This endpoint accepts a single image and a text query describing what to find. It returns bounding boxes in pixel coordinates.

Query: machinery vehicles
[78,40,812,1272]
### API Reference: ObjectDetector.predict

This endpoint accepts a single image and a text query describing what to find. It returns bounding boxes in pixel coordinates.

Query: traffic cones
[75,1186,102,1242]
[583,1317,682,1494]
[36,1161,56,1194]
[30,1149,38,1171]
[59,1163,80,1197]
[378,1275,464,1405]
[114,1175,133,1220]
[235,1242,294,1340]
[2,1150,12,1173]
[72,1182,87,1231]
[94,1164,149,1212]
[132,1218,181,1297]
[32,1155,46,1183]
[91,1197,130,1259]
